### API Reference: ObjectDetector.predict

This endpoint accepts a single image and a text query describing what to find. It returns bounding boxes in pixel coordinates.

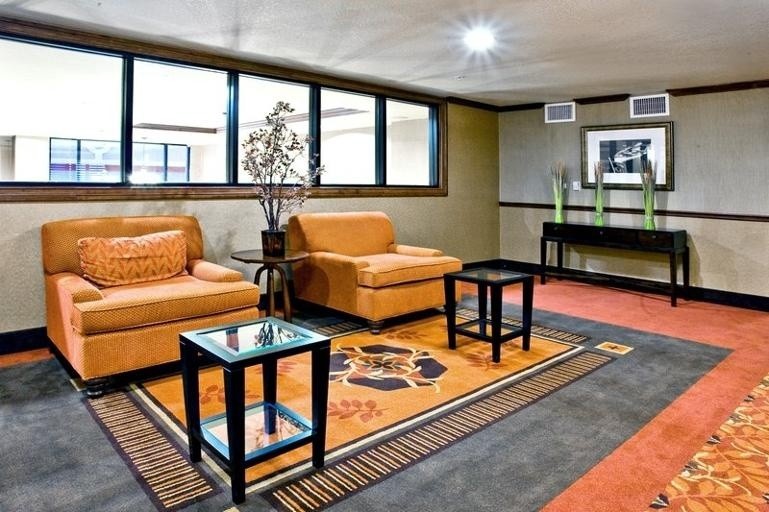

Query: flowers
[242,102,327,232]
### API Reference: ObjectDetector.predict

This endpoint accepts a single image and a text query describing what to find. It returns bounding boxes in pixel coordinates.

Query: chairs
[286,211,463,335]
[40,215,260,398]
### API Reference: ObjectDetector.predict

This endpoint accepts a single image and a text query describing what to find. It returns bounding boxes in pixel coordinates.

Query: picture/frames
[579,121,675,192]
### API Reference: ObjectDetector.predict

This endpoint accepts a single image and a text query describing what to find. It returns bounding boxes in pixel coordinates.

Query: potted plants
[594,161,604,227]
[639,160,658,231]
[547,158,571,224]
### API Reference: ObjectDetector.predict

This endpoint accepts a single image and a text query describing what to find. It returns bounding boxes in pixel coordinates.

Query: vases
[261,229,286,258]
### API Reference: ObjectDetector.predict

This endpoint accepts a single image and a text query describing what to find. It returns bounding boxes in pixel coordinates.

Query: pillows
[78,229,188,287]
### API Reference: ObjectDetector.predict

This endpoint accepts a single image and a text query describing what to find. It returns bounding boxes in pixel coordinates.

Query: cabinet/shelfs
[541,222,691,307]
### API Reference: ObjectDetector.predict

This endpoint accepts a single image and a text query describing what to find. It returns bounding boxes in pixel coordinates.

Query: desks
[232,249,310,324]
